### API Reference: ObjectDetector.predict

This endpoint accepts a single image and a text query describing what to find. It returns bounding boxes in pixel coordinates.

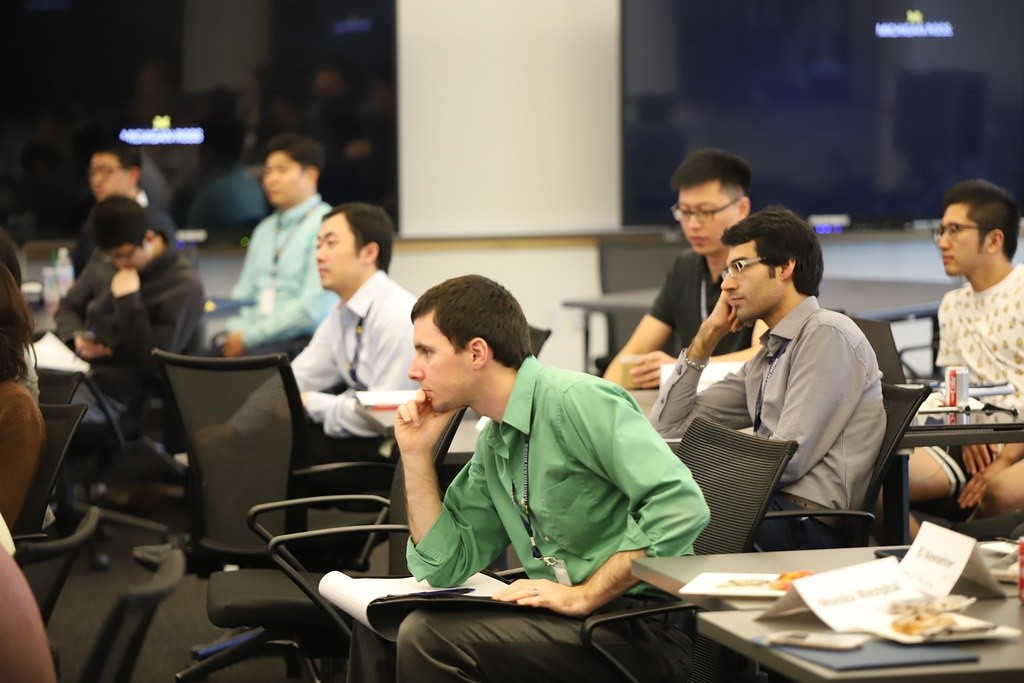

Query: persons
[872,177,1024,546]
[346,275,710,683]
[132,201,418,577]
[648,207,886,554]
[218,134,340,357]
[53,141,207,506]
[603,151,771,392]
[0,233,58,683]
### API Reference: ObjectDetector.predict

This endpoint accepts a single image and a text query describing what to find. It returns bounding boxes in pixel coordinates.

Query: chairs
[14,243,1024,683]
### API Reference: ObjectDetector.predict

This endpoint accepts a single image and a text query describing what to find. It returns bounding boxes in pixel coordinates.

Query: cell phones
[874,548,911,562]
[82,334,108,348]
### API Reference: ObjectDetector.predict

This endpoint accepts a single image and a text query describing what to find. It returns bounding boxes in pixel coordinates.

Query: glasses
[96,245,137,264]
[932,221,980,244]
[721,255,766,281]
[670,198,739,223]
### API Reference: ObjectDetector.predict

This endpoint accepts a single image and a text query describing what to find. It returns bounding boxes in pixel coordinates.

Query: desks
[630,540,1024,610]
[28,287,254,339]
[355,388,1024,470]
[561,276,984,380]
[692,595,1024,683]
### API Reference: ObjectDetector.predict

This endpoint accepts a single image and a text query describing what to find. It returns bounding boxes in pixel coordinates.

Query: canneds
[42,266,60,306]
[942,411,969,426]
[945,365,969,408]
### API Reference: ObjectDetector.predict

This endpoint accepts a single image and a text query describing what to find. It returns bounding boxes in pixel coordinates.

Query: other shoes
[135,534,221,579]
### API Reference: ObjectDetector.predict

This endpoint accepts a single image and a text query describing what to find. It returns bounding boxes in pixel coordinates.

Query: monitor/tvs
[0,0,400,247]
[621,0,1024,231]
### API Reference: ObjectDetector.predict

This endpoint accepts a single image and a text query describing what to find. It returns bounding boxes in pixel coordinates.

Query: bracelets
[683,349,710,370]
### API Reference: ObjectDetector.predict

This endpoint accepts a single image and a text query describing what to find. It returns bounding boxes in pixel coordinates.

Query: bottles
[42,247,74,314]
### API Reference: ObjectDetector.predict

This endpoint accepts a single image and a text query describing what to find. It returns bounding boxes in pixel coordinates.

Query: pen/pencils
[422,586,476,595]
[67,329,98,363]
